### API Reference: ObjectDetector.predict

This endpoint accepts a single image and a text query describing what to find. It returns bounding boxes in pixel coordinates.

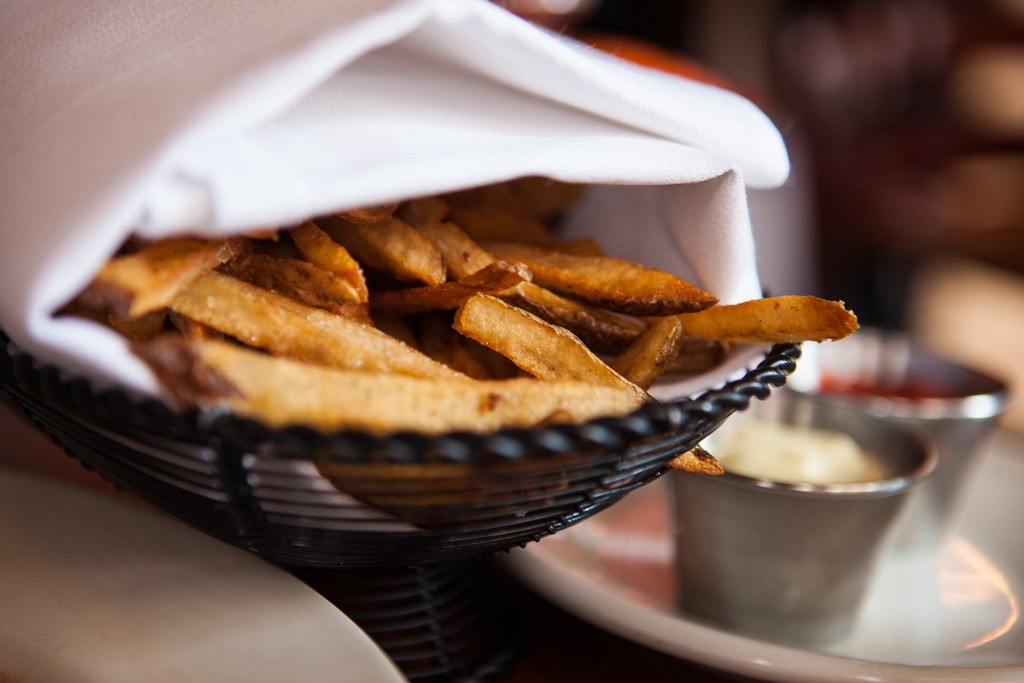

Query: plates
[507,468,1024,682]
[0,470,409,683]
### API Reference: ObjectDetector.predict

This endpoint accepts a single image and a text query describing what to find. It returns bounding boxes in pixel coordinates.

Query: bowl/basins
[779,359,1010,548]
[667,395,937,648]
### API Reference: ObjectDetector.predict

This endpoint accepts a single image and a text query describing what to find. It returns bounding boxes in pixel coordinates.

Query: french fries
[76,175,857,474]
